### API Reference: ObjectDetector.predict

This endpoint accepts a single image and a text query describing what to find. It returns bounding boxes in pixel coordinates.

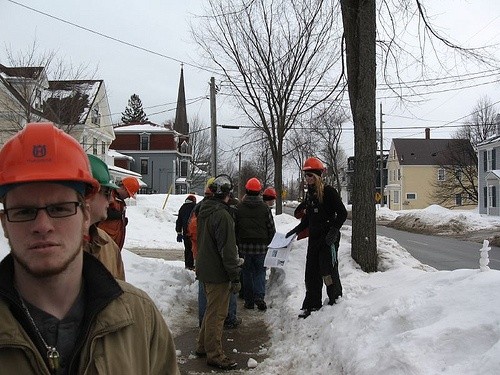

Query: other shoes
[194,351,207,358]
[185,266,196,270]
[231,280,241,295]
[224,317,243,329]
[298,308,321,318]
[207,357,237,369]
[328,297,338,305]
[244,298,267,310]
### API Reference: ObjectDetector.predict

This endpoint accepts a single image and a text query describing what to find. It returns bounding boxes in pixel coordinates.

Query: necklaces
[15,283,61,369]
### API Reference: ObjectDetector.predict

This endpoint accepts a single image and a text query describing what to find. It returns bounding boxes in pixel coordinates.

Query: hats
[204,174,234,198]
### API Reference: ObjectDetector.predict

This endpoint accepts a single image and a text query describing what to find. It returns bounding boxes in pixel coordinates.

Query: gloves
[326,225,338,247]
[177,232,184,242]
[285,225,301,238]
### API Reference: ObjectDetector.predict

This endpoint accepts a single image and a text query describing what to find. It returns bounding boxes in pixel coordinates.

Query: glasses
[4,201,81,222]
[103,189,111,198]
[304,173,314,178]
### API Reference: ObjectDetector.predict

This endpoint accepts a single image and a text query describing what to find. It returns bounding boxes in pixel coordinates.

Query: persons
[95,176,139,251]
[286,157,349,318]
[0,122,179,375]
[83,154,125,281]
[176,174,277,329]
[194,175,241,369]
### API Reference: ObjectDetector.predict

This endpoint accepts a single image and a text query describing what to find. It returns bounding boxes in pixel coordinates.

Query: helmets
[87,154,120,189]
[185,195,196,202]
[245,178,261,191]
[0,122,101,200]
[303,157,324,171]
[263,187,278,200]
[122,176,140,197]
